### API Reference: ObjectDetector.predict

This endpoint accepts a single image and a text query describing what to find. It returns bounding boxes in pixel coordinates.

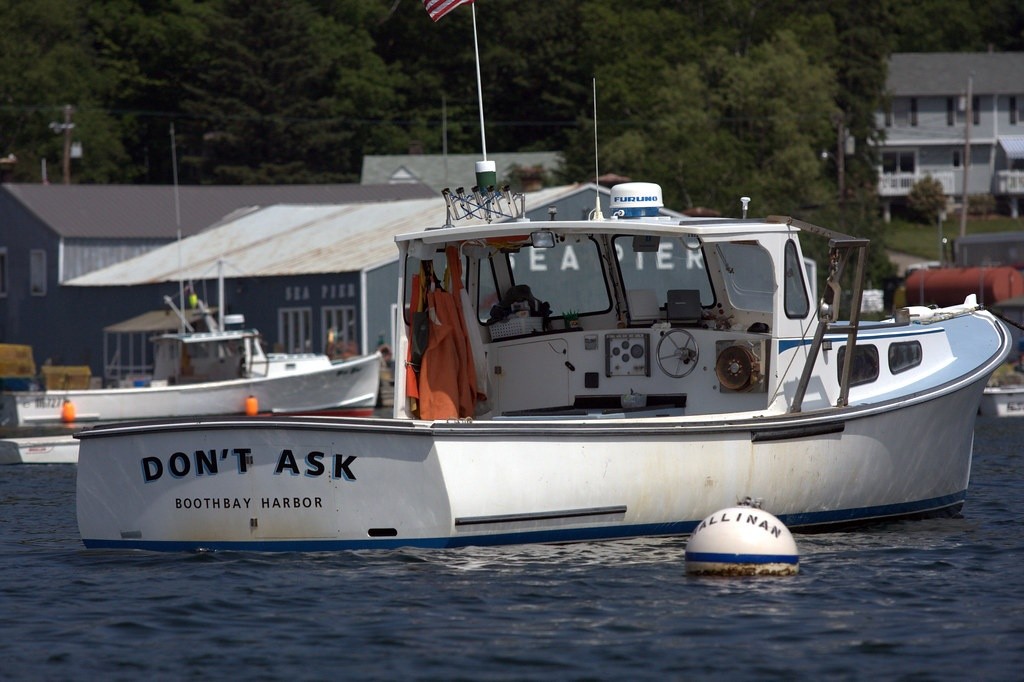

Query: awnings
[998,138,1024,159]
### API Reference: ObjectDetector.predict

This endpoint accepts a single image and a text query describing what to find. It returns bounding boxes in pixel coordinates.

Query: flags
[424,0,477,22]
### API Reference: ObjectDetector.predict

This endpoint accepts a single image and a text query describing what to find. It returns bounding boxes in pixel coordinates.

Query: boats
[979,386,1024,417]
[0,259,380,427]
[72,161,1011,551]
[0,434,81,466]
